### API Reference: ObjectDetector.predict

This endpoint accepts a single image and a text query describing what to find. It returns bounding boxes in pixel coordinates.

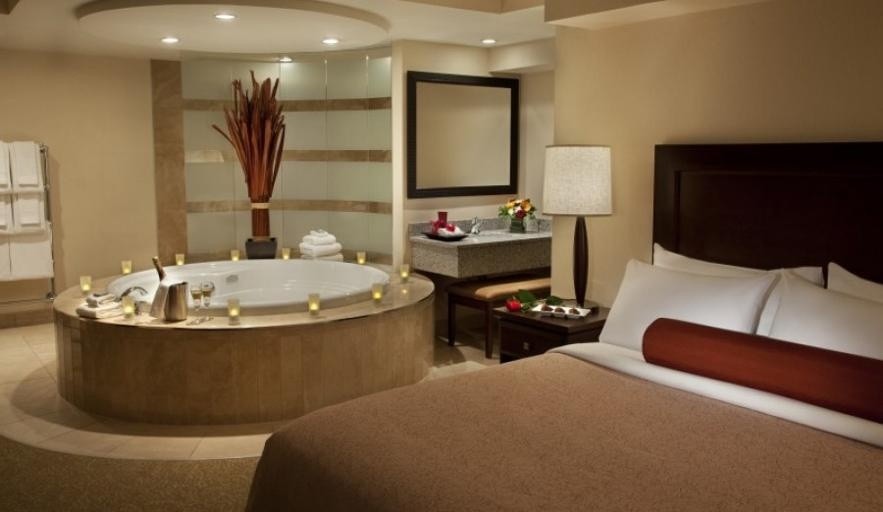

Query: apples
[505,296,520,313]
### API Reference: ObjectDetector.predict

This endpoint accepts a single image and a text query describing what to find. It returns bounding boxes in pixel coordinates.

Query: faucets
[116,286,149,303]
[471,214,489,235]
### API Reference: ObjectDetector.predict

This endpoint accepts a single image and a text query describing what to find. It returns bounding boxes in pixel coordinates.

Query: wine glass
[191,282,214,325]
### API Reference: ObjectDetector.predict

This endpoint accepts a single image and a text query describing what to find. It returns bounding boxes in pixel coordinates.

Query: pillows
[641,317,883,423]
[826,262,882,304]
[653,244,824,296]
[765,267,882,358]
[598,259,782,371]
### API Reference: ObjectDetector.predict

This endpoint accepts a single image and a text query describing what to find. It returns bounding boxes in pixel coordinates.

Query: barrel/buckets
[162,283,194,321]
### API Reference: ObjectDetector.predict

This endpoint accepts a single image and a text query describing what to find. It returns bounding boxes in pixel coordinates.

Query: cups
[356,252,366,264]
[121,260,132,274]
[176,253,185,265]
[231,249,240,260]
[282,248,290,259]
[399,264,409,283]
[372,283,383,303]
[79,275,92,296]
[122,296,135,319]
[308,293,320,317]
[228,298,241,325]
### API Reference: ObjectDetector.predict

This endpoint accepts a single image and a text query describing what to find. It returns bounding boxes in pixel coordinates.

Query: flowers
[499,197,536,220]
[506,290,566,315]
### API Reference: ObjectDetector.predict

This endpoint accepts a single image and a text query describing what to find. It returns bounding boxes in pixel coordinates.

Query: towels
[76,303,122,319]
[1,140,54,282]
[87,291,115,307]
[149,274,188,319]
[297,225,343,261]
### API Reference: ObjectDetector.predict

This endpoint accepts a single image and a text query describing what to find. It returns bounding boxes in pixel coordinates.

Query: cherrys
[446,224,455,232]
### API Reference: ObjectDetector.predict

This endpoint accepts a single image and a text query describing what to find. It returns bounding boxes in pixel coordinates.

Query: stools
[447,277,550,361]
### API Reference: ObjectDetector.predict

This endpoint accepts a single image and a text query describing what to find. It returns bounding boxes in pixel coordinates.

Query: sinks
[474,233,513,241]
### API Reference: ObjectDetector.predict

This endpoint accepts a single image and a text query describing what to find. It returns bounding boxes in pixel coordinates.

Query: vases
[509,218,523,231]
[245,237,278,260]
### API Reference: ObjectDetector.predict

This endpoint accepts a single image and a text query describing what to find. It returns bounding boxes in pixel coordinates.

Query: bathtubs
[106,256,391,315]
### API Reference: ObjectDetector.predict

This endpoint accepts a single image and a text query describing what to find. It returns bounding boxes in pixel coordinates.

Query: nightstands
[492,298,613,364]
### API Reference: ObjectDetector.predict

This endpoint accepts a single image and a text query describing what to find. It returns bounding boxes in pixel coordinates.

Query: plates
[531,305,591,320]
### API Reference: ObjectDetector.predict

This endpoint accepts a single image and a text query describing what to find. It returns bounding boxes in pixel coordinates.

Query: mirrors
[407,69,519,199]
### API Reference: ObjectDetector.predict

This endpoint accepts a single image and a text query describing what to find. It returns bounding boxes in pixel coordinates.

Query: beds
[244,141,883,511]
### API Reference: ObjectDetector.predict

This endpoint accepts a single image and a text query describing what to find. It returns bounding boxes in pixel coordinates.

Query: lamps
[544,147,613,313]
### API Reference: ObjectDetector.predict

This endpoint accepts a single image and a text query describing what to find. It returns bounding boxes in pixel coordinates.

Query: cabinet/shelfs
[1,140,67,330]
